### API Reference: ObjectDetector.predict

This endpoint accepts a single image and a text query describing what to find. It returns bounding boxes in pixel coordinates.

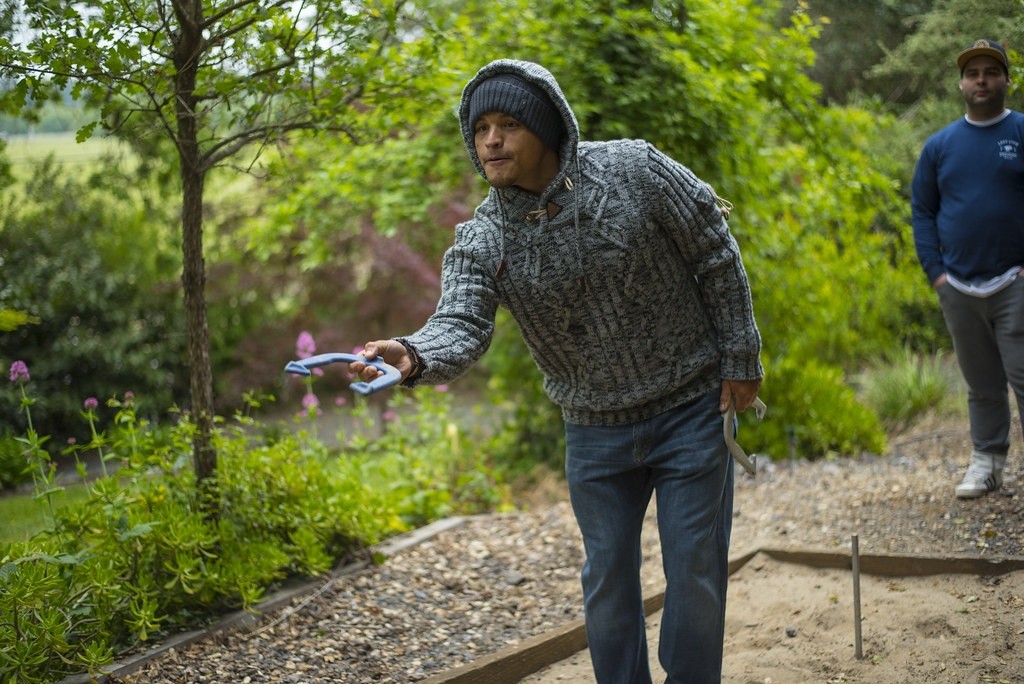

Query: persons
[912,38,1022,498]
[349,58,765,684]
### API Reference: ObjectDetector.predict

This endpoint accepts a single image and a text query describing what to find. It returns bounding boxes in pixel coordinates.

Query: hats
[469,73,560,153]
[957,38,1009,73]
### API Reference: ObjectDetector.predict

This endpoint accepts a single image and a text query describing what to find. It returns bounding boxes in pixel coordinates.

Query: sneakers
[956,452,1005,496]
